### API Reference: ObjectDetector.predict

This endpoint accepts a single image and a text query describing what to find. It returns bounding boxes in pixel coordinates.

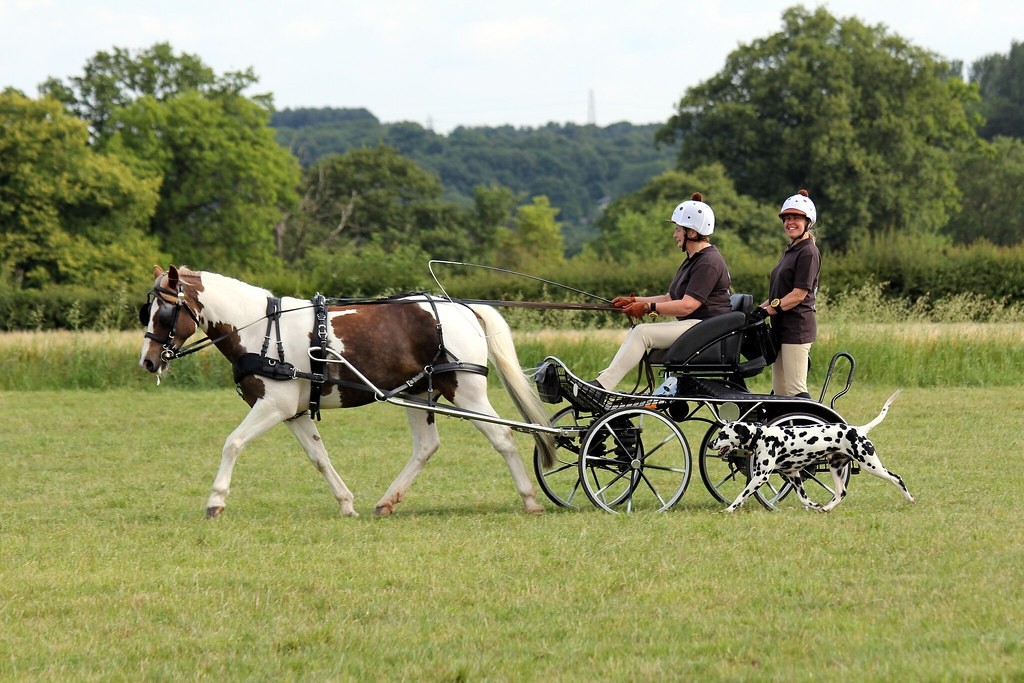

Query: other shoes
[561,380,607,412]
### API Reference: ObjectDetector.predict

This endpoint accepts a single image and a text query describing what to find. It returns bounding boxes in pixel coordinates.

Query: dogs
[706,386,917,515]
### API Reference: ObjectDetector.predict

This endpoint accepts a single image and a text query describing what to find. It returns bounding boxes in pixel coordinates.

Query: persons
[565,193,736,405]
[759,191,822,400]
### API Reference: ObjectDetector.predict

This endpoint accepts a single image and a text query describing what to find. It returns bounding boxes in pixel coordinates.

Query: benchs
[647,292,755,373]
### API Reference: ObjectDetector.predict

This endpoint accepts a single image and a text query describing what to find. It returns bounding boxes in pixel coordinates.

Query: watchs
[771,299,784,314]
[648,303,659,318]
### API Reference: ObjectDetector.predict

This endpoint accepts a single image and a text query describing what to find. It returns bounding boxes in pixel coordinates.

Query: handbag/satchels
[740,320,778,366]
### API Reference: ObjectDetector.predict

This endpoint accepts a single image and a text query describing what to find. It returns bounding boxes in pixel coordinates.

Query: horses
[138,262,559,515]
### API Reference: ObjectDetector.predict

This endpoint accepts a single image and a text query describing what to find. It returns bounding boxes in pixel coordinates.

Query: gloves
[748,306,770,325]
[621,301,650,318]
[610,293,636,308]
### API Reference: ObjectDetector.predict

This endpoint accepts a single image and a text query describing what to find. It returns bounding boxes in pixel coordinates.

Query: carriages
[132,261,858,519]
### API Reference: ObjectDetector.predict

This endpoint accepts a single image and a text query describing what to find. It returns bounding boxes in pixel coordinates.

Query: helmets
[778,189,816,229]
[665,192,716,236]
